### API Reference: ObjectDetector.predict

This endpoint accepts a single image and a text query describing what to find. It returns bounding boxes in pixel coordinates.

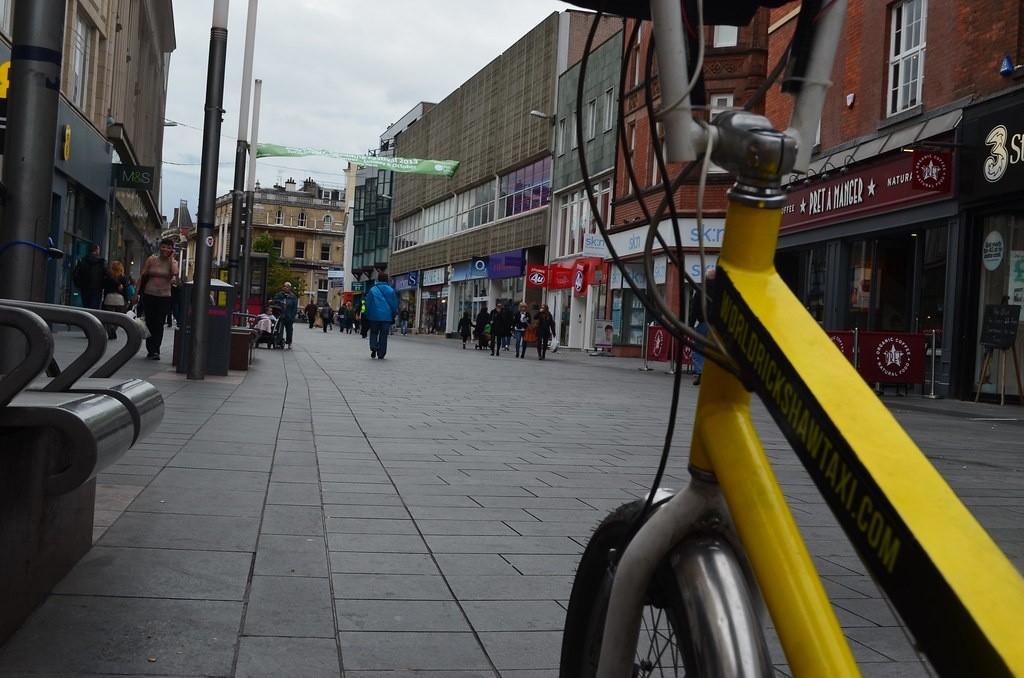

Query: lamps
[787,154,859,193]
[383,193,393,200]
[531,110,556,126]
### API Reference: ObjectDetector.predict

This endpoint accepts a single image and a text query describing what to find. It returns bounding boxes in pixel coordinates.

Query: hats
[705,268,716,279]
[519,302,527,311]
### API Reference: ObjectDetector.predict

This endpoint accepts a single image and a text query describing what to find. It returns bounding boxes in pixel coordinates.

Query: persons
[131,238,179,359]
[73,242,130,340]
[273,282,298,349]
[687,267,715,386]
[364,272,398,358]
[257,307,277,331]
[305,300,323,328]
[457,312,476,349]
[400,305,410,336]
[475,302,513,356]
[534,304,556,360]
[338,300,369,338]
[511,302,531,358]
[320,302,335,332]
[598,325,613,344]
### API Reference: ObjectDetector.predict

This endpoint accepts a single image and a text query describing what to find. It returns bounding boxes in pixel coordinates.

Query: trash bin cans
[177,278,234,376]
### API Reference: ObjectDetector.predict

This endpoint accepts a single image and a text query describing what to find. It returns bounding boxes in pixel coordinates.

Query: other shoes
[521,355,524,358]
[288,345,292,349]
[502,348,505,351]
[405,334,407,336]
[167,323,172,327]
[516,354,519,358]
[538,357,541,360]
[542,355,545,359]
[378,355,383,359]
[371,349,376,358]
[401,334,403,336]
[693,375,701,385]
[496,352,499,356]
[108,335,116,339]
[491,353,494,356]
[506,346,510,350]
[148,352,160,360]
[462,343,466,349]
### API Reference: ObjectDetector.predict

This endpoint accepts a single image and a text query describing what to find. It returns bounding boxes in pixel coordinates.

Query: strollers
[255,299,286,349]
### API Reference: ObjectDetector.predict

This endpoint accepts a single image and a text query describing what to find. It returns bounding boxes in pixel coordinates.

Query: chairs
[230,311,262,369]
[0,295,165,647]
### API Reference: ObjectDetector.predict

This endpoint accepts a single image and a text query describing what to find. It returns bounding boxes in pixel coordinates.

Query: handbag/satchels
[340,315,344,318]
[141,270,150,283]
[329,309,333,320]
[523,320,539,341]
[484,324,491,333]
[391,313,395,324]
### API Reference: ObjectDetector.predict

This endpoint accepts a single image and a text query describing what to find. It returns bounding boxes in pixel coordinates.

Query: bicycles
[561,0,1023,677]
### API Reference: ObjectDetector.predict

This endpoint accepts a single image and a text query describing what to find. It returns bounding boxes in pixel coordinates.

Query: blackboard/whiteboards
[979,304,1021,349]
